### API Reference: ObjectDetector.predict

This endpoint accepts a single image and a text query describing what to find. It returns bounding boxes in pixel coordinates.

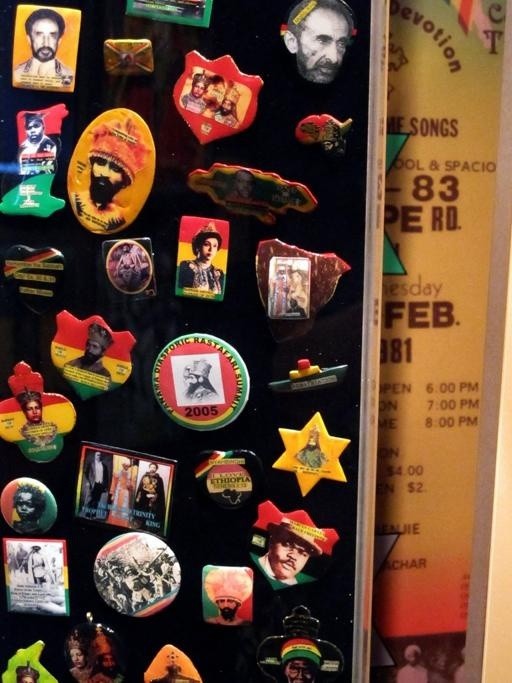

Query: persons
[395,644,455,683]
[8,1,353,683]
